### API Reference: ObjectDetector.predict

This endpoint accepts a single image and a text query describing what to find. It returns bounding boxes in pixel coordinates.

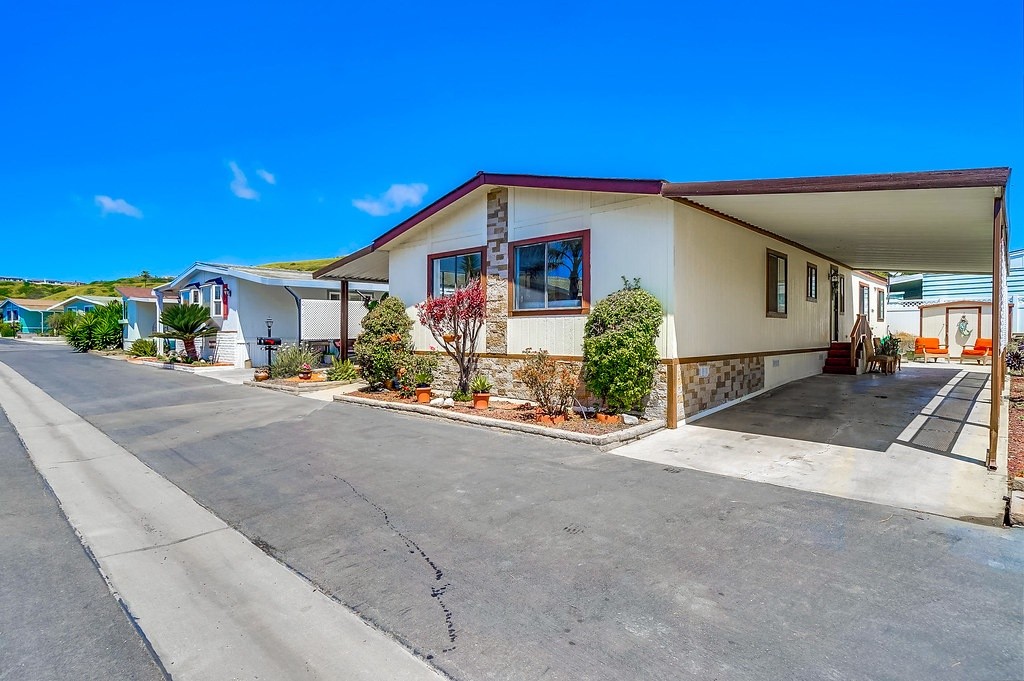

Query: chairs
[874,337,903,372]
[863,338,894,376]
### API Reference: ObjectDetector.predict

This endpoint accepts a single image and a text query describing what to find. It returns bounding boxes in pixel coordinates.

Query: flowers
[297,362,312,371]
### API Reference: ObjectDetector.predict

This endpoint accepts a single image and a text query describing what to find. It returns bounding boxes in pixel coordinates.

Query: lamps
[828,268,840,287]
[224,286,231,297]
[264,315,274,328]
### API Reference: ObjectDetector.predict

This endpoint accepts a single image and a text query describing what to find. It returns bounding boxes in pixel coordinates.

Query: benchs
[333,339,357,363]
[913,335,951,363]
[960,338,991,365]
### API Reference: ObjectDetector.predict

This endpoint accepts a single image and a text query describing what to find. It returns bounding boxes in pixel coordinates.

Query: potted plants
[469,369,495,409]
[414,367,432,402]
[299,369,314,380]
